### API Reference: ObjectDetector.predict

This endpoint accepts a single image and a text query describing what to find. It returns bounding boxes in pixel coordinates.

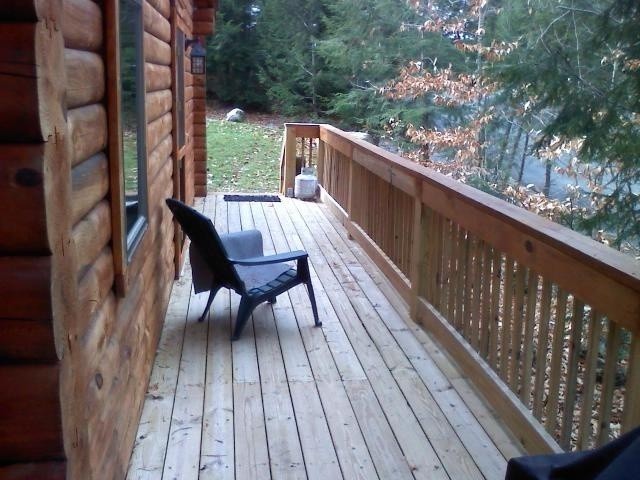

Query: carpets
[223,195,281,202]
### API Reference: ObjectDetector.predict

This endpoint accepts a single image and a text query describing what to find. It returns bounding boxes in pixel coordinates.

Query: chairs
[166,198,323,341]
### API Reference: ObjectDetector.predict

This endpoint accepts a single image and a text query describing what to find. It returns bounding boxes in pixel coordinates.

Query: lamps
[185,36,206,75]
